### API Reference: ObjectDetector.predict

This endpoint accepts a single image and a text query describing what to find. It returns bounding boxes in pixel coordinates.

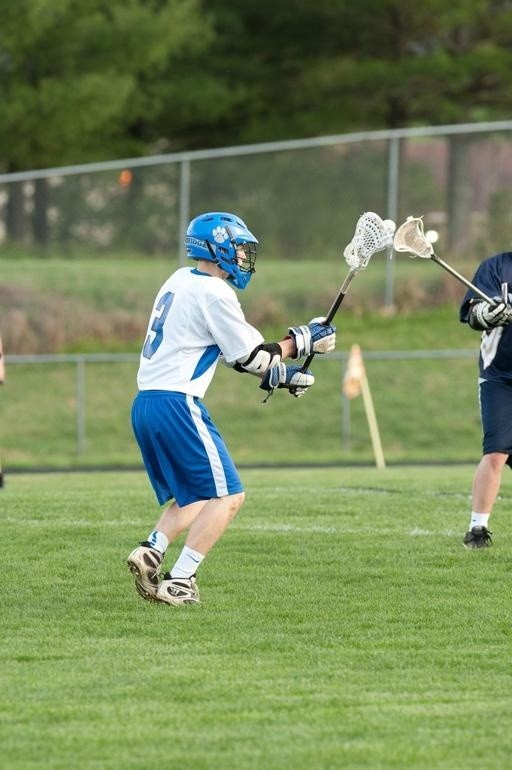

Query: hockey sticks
[393,216,499,308]
[288,211,393,395]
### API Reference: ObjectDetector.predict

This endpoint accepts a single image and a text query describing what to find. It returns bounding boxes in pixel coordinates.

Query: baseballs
[382,218,396,231]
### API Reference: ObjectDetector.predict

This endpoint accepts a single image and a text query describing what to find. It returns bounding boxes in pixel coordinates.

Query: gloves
[476,296,512,329]
[288,317,336,361]
[259,363,315,398]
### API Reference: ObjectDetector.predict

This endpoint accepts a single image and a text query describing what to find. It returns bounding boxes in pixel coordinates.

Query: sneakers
[127,541,164,601]
[156,572,200,606]
[463,526,493,549]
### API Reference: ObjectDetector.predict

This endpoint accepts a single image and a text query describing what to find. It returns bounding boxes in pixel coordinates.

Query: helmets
[185,212,259,288]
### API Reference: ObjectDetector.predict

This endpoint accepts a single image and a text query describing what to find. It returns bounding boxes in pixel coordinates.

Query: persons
[459,250,512,549]
[127,211,336,608]
